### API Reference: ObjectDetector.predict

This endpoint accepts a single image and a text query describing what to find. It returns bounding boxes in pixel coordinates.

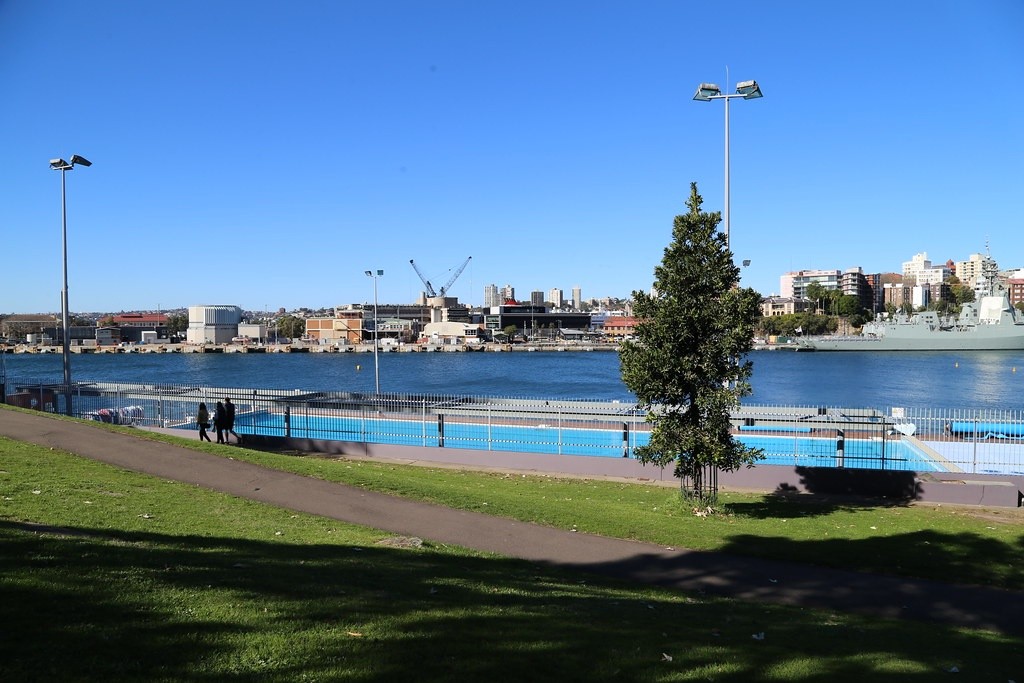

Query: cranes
[439,255,471,300]
[405,258,437,299]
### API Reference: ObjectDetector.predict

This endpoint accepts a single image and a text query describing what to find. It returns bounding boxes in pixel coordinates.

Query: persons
[196,402,212,443]
[213,397,241,444]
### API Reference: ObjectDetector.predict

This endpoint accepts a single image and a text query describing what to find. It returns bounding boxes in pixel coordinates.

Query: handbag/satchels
[205,422,210,428]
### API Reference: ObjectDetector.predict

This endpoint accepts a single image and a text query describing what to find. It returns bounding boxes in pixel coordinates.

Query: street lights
[364,269,384,396]
[693,80,762,401]
[50,155,91,385]
